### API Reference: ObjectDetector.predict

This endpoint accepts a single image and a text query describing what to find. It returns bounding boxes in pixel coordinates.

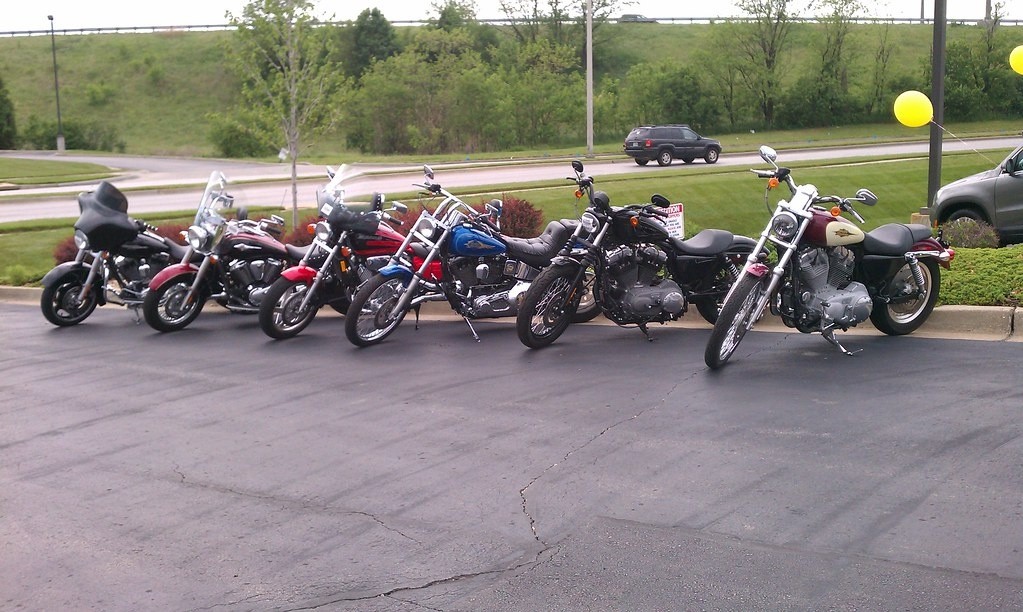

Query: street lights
[46,15,66,151]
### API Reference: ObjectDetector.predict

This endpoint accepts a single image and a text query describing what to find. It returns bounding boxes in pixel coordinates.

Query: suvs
[622,124,722,167]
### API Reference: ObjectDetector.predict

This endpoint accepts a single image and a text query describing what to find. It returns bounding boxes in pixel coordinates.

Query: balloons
[1009,45,1023,75]
[893,90,933,127]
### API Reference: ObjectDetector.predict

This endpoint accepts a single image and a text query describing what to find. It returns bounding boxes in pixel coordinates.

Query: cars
[617,13,659,24]
[927,143,1023,249]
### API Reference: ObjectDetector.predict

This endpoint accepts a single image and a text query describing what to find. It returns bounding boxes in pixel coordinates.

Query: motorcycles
[257,161,505,341]
[37,180,286,327]
[141,170,386,333]
[704,145,957,370]
[342,163,603,349]
[515,160,771,349]
